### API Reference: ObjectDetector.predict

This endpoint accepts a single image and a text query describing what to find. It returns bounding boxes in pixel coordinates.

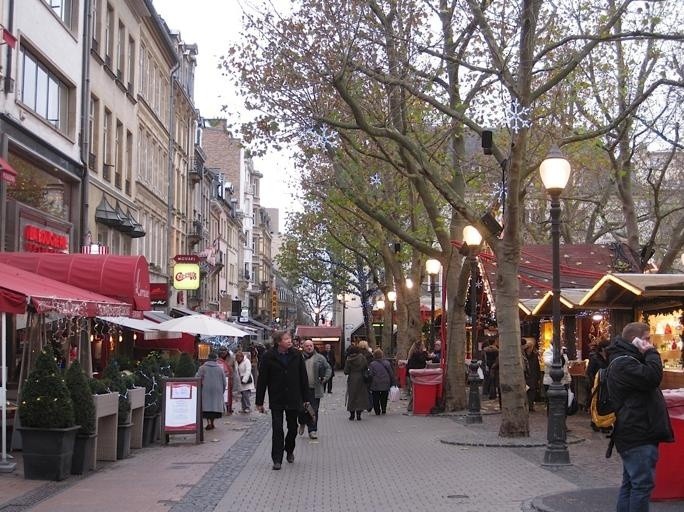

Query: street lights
[422,247,440,354]
[362,276,423,358]
[535,138,576,469]
[336,291,350,369]
[313,307,320,326]
[461,220,485,423]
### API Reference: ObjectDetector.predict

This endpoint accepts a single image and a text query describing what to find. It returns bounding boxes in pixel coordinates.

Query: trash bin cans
[647,387,684,502]
[408,368,443,417]
[398,359,408,388]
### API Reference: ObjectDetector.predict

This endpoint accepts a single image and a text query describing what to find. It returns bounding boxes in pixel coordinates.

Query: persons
[542,338,573,431]
[586,340,614,433]
[607,322,674,512]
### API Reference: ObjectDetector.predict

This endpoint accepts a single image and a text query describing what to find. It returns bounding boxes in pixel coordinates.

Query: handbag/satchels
[241,375,252,384]
[298,403,316,424]
[320,365,325,380]
[363,367,369,382]
[367,390,373,412]
[567,390,578,415]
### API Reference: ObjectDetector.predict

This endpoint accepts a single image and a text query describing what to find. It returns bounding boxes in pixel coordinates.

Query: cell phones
[633,338,642,347]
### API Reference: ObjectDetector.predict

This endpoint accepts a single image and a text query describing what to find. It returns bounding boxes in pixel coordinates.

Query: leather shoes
[310,431,317,439]
[357,415,361,420]
[206,425,214,429]
[376,412,380,415]
[349,415,355,420]
[274,463,281,469]
[382,404,386,413]
[287,452,294,462]
[299,425,304,435]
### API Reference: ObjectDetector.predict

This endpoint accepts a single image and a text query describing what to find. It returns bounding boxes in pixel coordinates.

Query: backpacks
[591,356,641,433]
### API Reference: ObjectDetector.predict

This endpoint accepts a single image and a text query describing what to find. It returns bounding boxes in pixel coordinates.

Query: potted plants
[12,343,198,481]
